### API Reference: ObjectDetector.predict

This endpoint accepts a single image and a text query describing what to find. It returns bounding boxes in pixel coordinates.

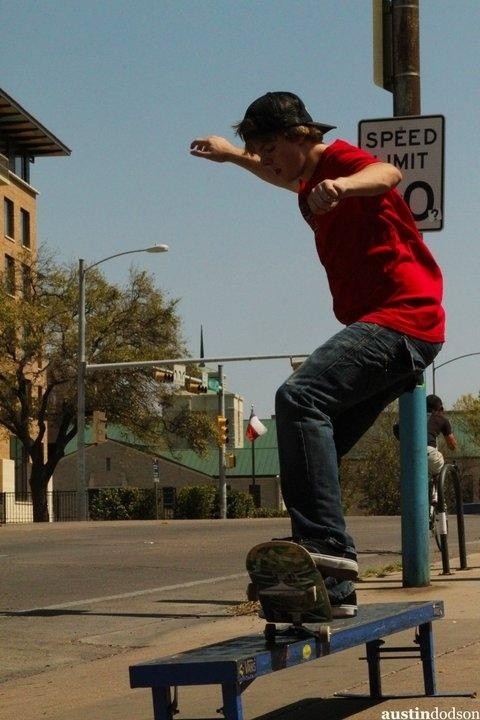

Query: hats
[241,91,338,142]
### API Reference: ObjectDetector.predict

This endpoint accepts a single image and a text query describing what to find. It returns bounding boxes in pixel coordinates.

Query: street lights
[77,244,169,521]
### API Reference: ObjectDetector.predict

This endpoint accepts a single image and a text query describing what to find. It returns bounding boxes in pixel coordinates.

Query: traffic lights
[216,415,229,447]
[224,453,236,469]
[185,383,207,394]
[154,370,174,384]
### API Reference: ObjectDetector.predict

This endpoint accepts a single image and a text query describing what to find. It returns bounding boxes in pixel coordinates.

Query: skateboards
[246,541,332,644]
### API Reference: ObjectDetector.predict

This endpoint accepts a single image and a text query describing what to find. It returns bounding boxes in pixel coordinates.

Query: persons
[393,394,457,531]
[189,90,445,621]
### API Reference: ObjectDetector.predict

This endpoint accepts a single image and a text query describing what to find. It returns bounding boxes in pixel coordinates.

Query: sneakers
[269,535,359,581]
[328,588,359,617]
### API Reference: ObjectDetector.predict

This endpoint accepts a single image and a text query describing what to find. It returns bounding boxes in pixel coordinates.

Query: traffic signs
[358,114,444,232]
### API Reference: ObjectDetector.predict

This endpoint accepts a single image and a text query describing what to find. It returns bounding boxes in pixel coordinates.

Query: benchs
[129,599,446,719]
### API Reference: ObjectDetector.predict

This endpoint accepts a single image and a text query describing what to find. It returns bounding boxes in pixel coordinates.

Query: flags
[246,408,268,442]
